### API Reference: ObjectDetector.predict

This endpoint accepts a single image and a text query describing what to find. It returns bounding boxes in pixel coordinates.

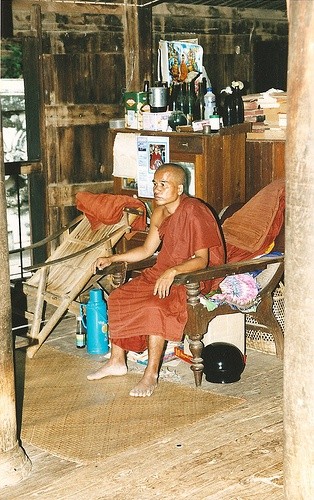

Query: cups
[148,86,167,113]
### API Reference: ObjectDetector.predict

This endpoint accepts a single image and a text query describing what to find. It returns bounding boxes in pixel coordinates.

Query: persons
[86,163,225,397]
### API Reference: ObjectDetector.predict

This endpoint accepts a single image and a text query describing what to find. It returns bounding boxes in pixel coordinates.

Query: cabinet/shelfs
[107,120,252,213]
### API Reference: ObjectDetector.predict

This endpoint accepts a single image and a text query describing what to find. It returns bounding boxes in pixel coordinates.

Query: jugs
[79,288,109,355]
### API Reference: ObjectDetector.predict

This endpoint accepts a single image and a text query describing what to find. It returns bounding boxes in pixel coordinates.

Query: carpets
[13,346,246,465]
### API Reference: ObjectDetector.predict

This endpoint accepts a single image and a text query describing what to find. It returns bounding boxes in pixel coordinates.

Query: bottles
[169,82,197,125]
[233,86,244,124]
[143,81,150,92]
[203,87,215,120]
[223,87,237,127]
[76,316,87,349]
[166,111,187,131]
[194,83,206,120]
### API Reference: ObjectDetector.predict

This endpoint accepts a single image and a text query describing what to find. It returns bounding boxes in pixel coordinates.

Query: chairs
[9,198,145,360]
[96,176,286,385]
